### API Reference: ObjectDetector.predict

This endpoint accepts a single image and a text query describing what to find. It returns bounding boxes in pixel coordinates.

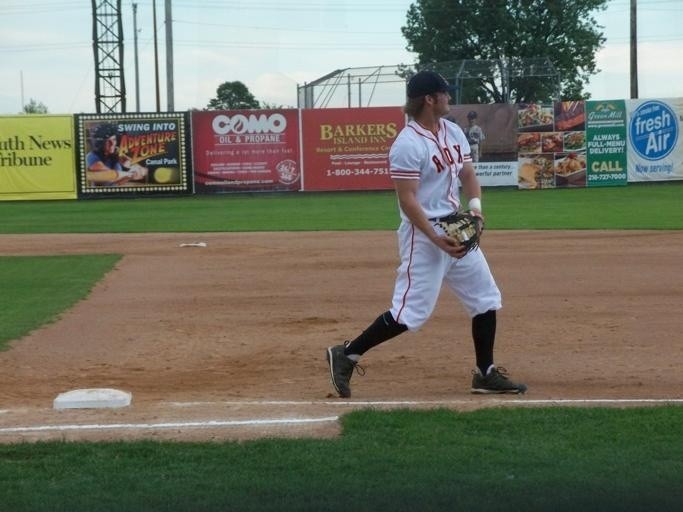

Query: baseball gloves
[433,210,479,252]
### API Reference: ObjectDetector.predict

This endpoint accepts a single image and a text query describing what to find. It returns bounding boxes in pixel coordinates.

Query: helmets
[89,122,116,153]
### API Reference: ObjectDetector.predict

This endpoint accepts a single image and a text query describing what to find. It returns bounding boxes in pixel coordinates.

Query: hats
[467,111,476,116]
[407,71,457,98]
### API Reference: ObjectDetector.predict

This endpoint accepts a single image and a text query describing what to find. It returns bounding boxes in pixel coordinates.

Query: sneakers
[327,341,365,397]
[470,365,528,394]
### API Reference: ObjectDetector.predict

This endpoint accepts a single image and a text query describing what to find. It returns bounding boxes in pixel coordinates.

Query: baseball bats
[86,170,137,183]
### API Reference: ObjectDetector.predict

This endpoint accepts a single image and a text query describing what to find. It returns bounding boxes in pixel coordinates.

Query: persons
[462,110,485,163]
[83,120,149,187]
[324,69,527,399]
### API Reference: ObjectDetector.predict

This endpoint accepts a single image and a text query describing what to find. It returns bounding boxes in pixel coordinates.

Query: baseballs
[155,168,170,183]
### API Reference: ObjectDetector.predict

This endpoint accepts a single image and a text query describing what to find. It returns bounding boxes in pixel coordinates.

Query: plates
[553,154,587,181]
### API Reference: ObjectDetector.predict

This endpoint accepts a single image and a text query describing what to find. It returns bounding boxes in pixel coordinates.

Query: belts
[429,218,447,222]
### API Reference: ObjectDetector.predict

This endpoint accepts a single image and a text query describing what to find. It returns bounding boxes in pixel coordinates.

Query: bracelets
[467,197,483,211]
